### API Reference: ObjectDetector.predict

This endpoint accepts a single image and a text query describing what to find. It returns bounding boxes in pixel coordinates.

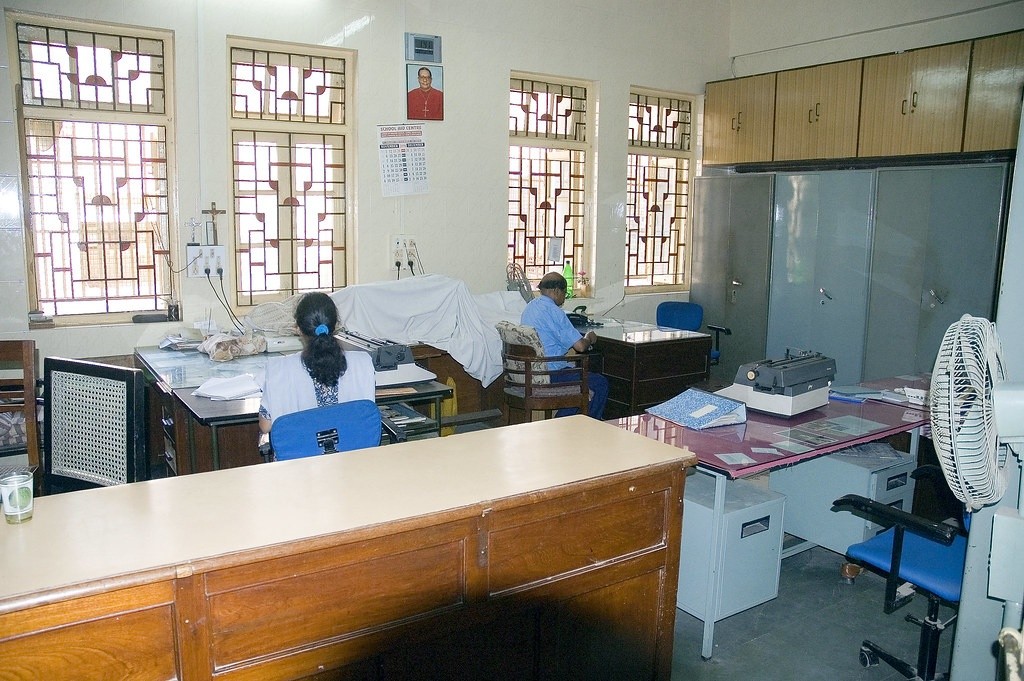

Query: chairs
[828,465,971,681]
[0,337,40,466]
[258,397,407,464]
[656,301,733,368]
[501,344,590,427]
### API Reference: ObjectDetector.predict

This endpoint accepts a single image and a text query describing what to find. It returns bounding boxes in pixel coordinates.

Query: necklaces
[421,89,431,116]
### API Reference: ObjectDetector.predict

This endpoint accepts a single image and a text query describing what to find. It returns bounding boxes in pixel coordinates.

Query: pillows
[495,321,550,386]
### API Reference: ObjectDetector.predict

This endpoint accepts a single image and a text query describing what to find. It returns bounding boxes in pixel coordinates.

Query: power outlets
[185,246,229,277]
[391,236,418,269]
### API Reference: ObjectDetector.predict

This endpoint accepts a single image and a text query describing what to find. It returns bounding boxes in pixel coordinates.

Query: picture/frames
[405,63,445,121]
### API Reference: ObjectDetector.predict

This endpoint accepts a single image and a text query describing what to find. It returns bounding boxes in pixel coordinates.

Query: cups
[168,304,179,321]
[0,471,34,525]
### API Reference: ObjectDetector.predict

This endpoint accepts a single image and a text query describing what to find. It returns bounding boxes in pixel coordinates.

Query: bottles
[561,260,573,299]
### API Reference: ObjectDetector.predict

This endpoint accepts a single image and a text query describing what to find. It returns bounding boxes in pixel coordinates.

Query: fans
[931,313,1024,681]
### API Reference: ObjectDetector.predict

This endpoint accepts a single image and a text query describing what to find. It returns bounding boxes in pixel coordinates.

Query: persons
[520,271,609,420]
[407,67,443,118]
[258,291,377,435]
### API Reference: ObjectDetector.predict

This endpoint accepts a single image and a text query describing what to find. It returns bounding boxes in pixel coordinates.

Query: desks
[595,372,935,659]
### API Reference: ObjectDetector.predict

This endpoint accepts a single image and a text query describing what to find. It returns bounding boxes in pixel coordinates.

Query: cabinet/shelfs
[0,414,701,679]
[136,342,501,477]
[700,28,1024,173]
[569,315,711,419]
[689,163,1010,388]
